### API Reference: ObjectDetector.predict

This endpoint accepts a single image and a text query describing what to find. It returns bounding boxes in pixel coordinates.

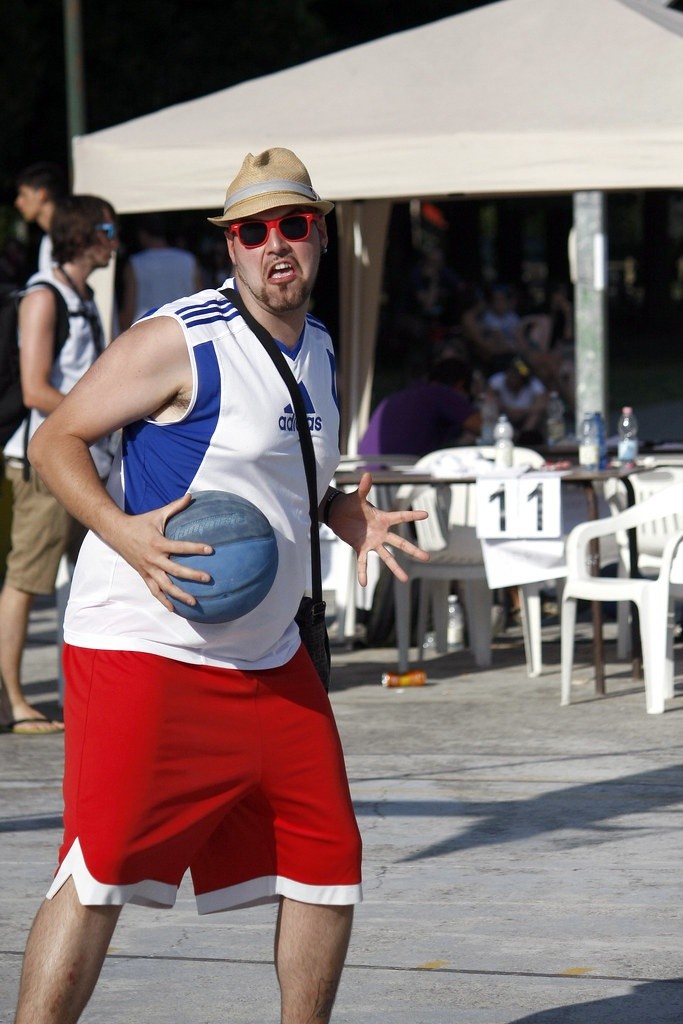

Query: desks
[524,464,662,696]
[333,455,476,648]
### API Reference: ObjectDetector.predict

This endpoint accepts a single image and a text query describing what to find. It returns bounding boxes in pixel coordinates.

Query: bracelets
[324,490,344,526]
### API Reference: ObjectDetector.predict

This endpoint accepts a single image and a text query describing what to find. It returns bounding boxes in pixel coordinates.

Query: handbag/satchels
[294,596,331,695]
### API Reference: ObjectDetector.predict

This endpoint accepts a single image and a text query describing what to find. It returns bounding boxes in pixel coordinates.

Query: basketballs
[157,490,279,625]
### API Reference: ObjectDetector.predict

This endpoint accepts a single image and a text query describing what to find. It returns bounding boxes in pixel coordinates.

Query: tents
[72,0,683,640]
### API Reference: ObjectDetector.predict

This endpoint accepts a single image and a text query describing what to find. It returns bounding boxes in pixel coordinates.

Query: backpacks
[0,281,69,451]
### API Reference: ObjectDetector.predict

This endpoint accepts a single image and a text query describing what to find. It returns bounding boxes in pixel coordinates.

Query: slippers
[0,717,64,735]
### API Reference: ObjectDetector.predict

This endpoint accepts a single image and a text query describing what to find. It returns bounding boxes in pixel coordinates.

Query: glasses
[95,223,117,241]
[228,213,320,248]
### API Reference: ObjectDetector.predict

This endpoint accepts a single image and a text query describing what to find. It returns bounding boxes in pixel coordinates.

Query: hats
[207,148,335,227]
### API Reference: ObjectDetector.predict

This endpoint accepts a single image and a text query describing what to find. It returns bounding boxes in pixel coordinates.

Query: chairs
[325,445,683,713]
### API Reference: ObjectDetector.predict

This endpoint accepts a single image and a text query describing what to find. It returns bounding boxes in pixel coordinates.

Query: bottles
[592,414,606,469]
[493,414,514,473]
[617,406,639,467]
[578,413,602,472]
[544,391,567,446]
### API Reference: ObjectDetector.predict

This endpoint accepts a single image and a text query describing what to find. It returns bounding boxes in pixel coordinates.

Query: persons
[357,227,580,641]
[14,147,430,1024]
[0,159,204,736]
